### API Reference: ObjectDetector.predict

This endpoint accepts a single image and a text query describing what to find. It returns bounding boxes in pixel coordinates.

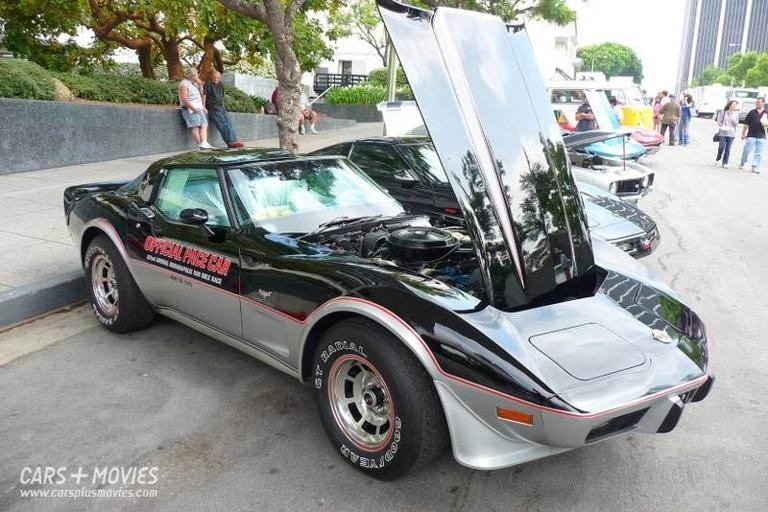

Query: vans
[682,82,768,121]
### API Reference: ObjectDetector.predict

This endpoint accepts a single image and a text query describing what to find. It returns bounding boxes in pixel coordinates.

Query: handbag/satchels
[689,109,696,116]
[713,132,719,141]
[265,105,277,114]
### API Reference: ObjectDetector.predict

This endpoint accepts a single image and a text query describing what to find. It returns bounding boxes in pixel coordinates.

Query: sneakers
[228,141,243,148]
[716,160,760,174]
[300,127,319,135]
[199,142,214,150]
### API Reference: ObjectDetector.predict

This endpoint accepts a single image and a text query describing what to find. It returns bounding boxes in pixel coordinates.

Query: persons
[297,84,318,135]
[203,70,243,149]
[608,95,625,125]
[178,67,214,150]
[654,90,767,174]
[574,97,593,131]
